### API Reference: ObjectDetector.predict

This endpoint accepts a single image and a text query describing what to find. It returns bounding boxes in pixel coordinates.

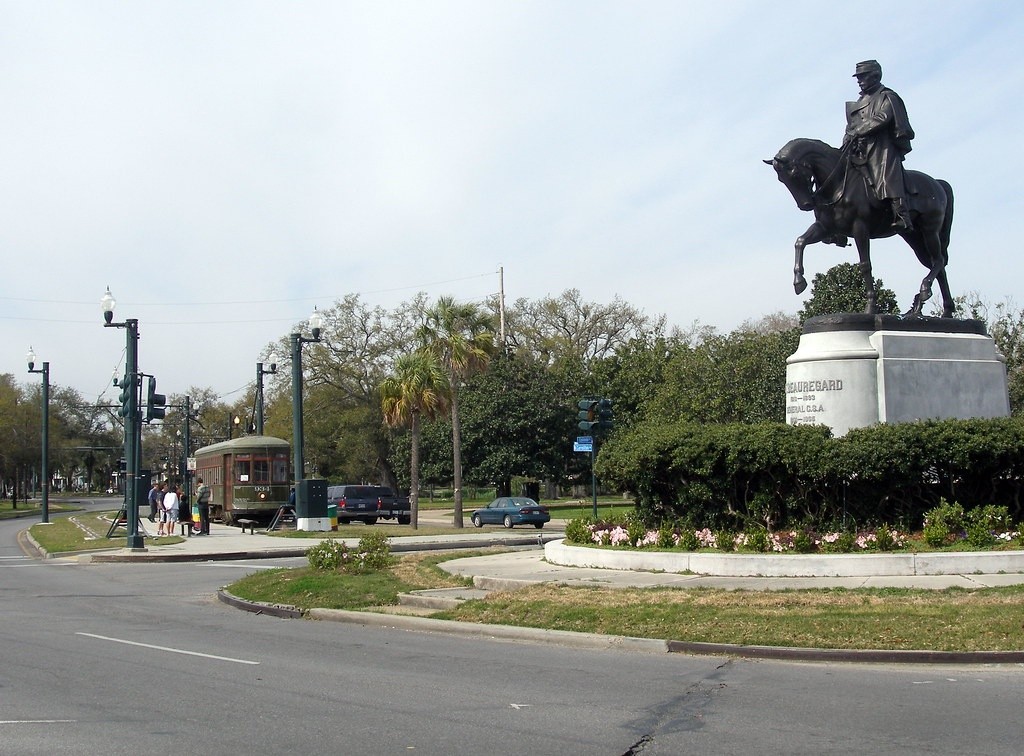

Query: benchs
[238,519,258,535]
[178,522,194,536]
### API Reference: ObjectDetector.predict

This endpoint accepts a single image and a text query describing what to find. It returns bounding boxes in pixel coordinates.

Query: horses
[762,137,958,322]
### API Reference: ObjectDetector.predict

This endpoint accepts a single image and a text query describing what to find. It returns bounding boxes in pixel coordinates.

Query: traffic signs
[573,435,593,452]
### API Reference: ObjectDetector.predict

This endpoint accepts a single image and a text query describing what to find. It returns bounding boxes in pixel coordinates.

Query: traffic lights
[146,377,166,421]
[599,398,614,429]
[577,399,599,432]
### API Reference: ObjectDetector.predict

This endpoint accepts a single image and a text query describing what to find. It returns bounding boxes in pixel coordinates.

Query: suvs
[326,484,383,525]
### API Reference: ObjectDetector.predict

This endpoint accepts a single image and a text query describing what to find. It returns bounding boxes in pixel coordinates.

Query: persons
[195,477,211,536]
[289,488,296,505]
[842,59,915,233]
[192,503,201,531]
[178,495,195,534]
[148,482,181,537]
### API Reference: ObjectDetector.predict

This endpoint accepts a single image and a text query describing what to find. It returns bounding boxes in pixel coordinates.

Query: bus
[189,435,291,527]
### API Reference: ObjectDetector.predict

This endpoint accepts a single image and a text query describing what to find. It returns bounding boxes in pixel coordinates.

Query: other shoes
[191,531,195,534]
[167,533,170,536]
[197,532,205,536]
[194,526,200,531]
[170,533,177,536]
[157,530,160,535]
[160,530,167,535]
[151,519,157,523]
[148,516,153,522]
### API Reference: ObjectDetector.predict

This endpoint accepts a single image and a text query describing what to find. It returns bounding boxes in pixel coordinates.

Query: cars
[471,497,550,529]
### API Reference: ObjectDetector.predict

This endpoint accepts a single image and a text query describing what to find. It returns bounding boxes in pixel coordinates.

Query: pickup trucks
[383,485,412,525]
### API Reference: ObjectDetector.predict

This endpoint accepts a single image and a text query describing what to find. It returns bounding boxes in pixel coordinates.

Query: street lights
[179,395,205,506]
[24,344,50,523]
[289,303,324,480]
[226,412,241,440]
[254,348,278,436]
[99,284,166,549]
